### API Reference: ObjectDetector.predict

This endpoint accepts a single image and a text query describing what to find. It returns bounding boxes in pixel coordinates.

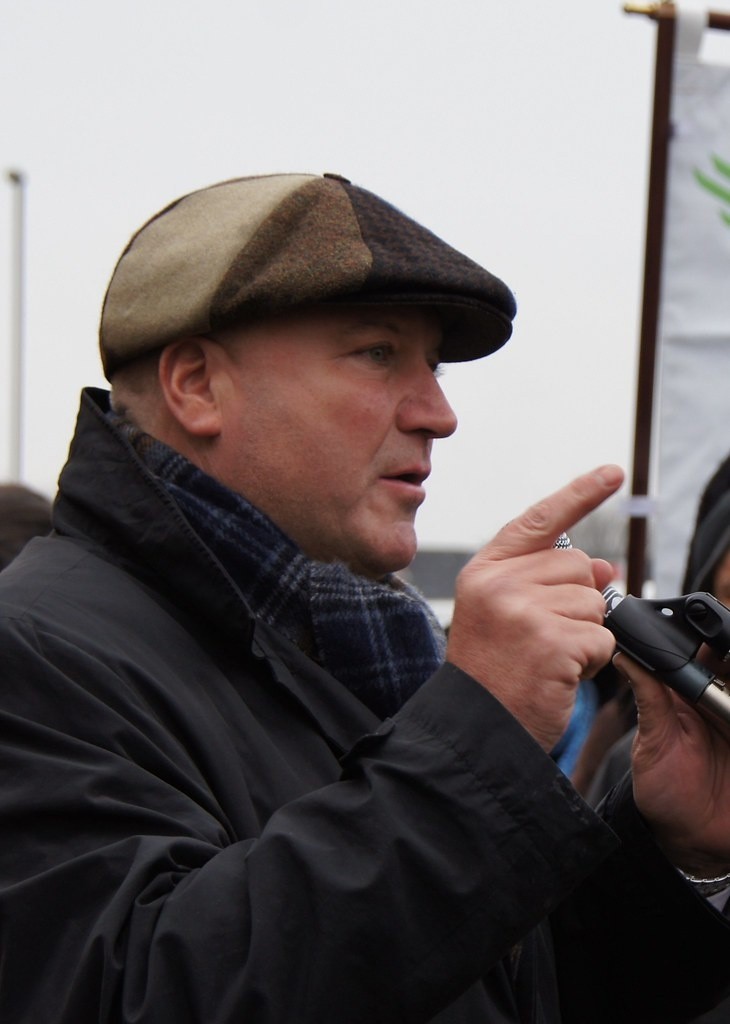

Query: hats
[99,173,518,384]
[682,455,730,595]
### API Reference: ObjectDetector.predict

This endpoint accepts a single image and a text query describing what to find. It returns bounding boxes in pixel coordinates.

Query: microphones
[552,535,730,744]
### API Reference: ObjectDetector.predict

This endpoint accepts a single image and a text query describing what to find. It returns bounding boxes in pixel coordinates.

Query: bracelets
[678,870,730,893]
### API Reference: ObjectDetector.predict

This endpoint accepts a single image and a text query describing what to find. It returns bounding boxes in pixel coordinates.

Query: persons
[0,172,730,1024]
[568,453,730,811]
